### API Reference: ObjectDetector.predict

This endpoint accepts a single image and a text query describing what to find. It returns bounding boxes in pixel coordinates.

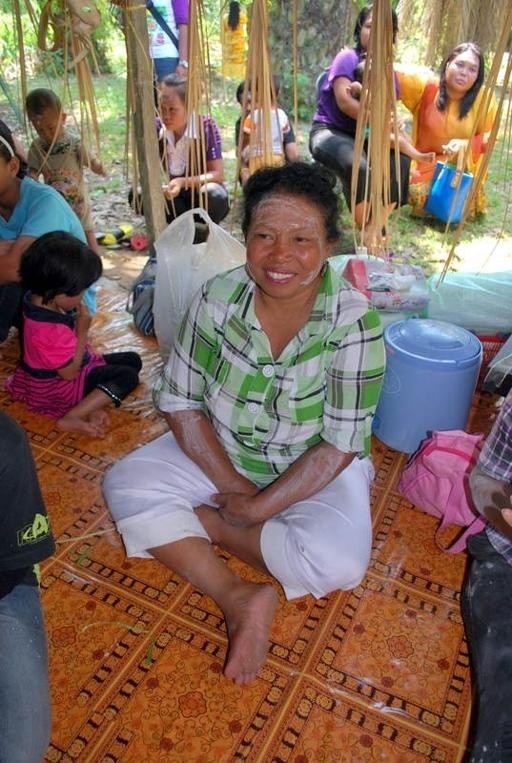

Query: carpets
[1,283,502,762]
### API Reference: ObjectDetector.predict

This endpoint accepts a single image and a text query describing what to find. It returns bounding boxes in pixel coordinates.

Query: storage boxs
[470,332,505,390]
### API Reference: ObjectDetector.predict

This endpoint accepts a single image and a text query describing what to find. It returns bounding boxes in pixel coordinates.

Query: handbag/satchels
[399,430,490,556]
[126,257,160,336]
[423,140,474,224]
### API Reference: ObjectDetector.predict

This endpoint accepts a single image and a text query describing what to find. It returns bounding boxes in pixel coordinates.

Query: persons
[0,411,57,763]
[0,119,98,343]
[146,1,190,111]
[235,78,298,194]
[308,4,412,255]
[380,42,502,225]
[24,88,109,259]
[7,230,142,439]
[219,0,250,79]
[101,159,387,687]
[460,390,512,763]
[346,58,436,164]
[237,74,290,172]
[128,74,230,225]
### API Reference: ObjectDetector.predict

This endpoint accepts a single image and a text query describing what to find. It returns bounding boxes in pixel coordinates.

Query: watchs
[178,60,189,69]
